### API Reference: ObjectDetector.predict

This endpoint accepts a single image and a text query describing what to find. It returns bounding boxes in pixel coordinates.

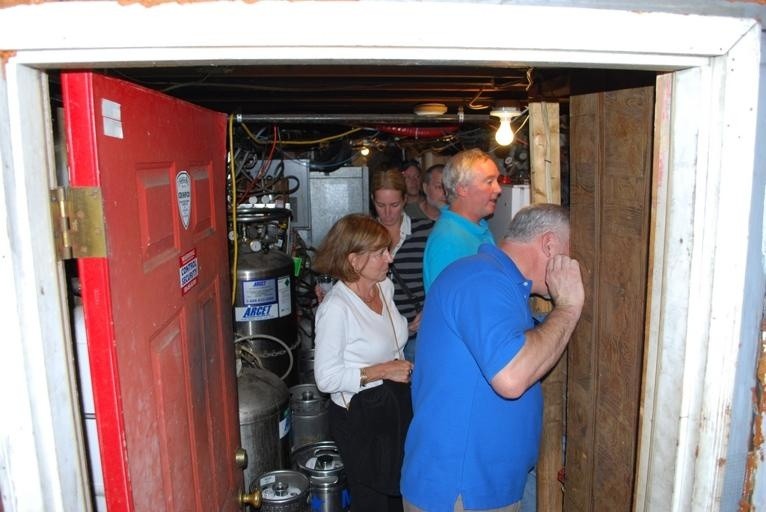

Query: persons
[308,146,585,512]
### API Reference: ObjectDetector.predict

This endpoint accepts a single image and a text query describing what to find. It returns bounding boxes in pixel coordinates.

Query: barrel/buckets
[301,441,353,511]
[248,470,309,512]
[291,383,329,450]
